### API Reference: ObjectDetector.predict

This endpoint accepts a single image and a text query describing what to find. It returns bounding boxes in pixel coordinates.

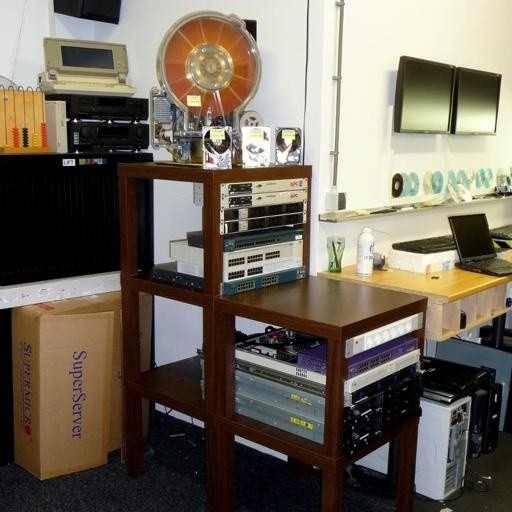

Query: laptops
[421,357,487,404]
[448,213,512,277]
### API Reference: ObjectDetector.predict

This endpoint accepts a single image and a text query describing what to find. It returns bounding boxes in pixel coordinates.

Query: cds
[447,184,460,203]
[456,184,473,202]
[392,166,512,197]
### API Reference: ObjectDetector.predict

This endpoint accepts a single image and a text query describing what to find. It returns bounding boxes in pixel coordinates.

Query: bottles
[356,227,375,278]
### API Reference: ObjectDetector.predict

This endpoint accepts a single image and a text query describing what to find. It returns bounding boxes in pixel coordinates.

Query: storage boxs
[11,285,154,480]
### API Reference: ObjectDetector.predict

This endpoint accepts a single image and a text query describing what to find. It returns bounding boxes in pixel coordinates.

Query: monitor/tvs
[395,55,456,134]
[451,66,502,135]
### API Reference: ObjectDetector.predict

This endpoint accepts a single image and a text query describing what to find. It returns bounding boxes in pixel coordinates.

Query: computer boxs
[354,380,503,505]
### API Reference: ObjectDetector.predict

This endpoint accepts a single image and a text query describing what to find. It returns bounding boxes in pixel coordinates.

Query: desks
[317,240,512,452]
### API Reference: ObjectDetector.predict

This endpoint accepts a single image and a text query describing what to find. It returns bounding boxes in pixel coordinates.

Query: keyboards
[391,234,457,254]
[488,224,512,239]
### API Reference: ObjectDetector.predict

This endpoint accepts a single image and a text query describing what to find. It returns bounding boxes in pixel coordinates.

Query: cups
[325,236,345,273]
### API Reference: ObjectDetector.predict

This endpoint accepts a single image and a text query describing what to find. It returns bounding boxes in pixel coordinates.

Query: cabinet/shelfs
[117,162,428,511]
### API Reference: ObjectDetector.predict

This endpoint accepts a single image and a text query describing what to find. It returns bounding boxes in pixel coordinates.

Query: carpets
[1,409,396,512]
